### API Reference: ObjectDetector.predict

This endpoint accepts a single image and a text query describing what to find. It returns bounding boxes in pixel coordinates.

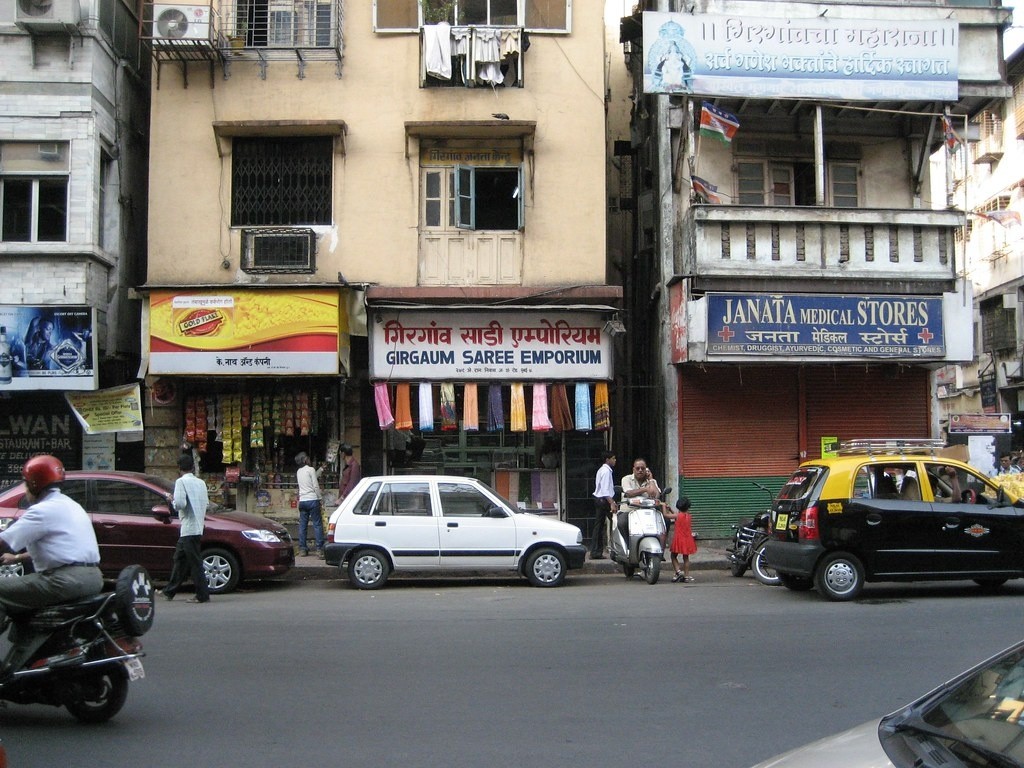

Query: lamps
[602,312,627,338]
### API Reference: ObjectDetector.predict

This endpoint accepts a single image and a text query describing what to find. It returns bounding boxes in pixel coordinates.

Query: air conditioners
[15,0,83,31]
[251,233,314,272]
[153,3,206,52]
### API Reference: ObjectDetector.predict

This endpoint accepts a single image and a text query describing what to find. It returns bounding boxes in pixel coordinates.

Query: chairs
[881,477,898,499]
[900,476,919,500]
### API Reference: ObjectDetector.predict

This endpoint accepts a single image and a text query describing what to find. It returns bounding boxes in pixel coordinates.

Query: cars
[751,640,1023,768]
[0,471,297,592]
[760,437,1024,602]
[319,474,586,591]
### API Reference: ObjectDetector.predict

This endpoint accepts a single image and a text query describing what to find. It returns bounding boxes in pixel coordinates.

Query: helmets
[22,455,65,496]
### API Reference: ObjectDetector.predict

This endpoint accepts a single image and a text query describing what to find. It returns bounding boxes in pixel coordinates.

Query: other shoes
[156,589,173,600]
[590,554,607,560]
[317,551,325,559]
[300,549,308,556]
[187,597,210,603]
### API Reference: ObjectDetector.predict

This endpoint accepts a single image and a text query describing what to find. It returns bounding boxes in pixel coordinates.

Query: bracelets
[341,496,344,500]
[15,554,23,566]
[614,501,615,503]
[649,480,654,482]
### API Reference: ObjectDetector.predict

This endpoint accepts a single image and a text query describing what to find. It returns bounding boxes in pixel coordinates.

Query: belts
[72,562,99,567]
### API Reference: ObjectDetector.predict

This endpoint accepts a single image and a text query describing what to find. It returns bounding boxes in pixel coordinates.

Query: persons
[616,458,671,561]
[992,447,1024,477]
[867,468,952,499]
[155,454,211,603]
[928,466,960,503]
[660,497,696,582]
[11,315,93,376]
[335,444,360,508]
[295,452,327,559]
[0,455,103,624]
[589,450,618,560]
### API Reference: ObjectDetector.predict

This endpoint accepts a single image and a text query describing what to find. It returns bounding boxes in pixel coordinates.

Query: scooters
[0,549,156,725]
[611,483,674,583]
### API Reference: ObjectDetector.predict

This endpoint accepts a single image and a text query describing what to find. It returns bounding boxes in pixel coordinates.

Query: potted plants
[225,22,250,56]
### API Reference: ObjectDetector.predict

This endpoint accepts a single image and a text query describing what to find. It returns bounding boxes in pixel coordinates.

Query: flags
[977,211,1021,224]
[699,100,740,149]
[940,113,955,149]
[691,176,720,204]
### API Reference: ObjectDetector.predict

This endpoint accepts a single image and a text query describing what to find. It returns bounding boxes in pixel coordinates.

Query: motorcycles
[724,481,786,586]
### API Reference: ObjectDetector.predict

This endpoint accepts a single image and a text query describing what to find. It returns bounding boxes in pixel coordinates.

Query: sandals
[672,570,683,582]
[684,575,694,582]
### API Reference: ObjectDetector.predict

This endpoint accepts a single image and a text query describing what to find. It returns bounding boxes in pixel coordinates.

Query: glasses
[636,466,645,470]
